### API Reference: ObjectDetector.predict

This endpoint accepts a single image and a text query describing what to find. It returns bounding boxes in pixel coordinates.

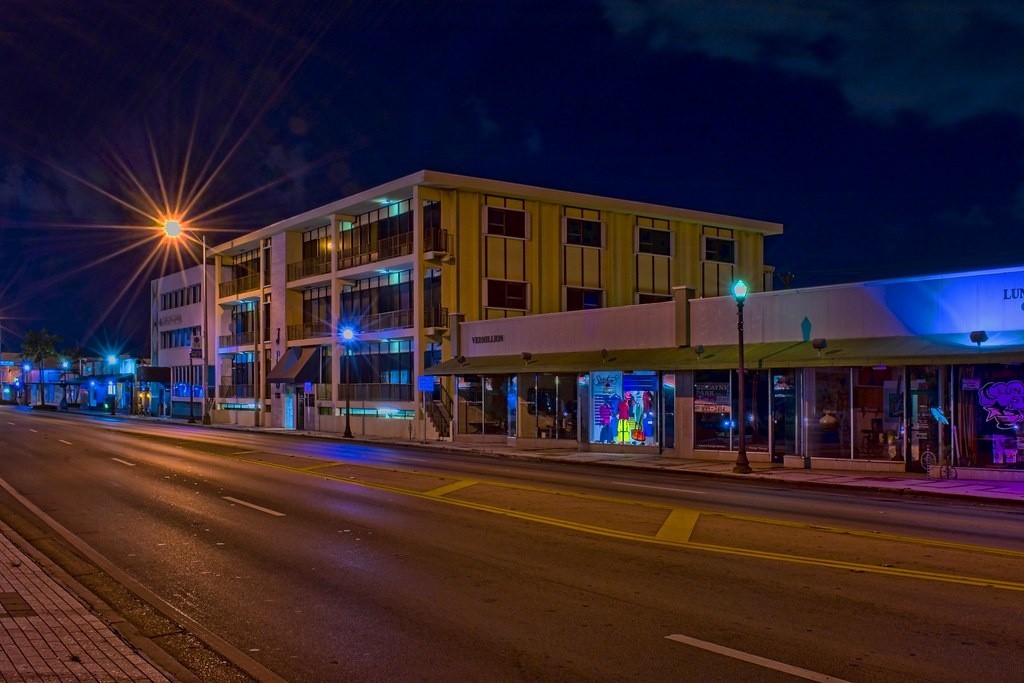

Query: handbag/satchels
[631,423,646,441]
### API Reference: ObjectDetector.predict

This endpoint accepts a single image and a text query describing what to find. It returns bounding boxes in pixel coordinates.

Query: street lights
[341,327,354,437]
[24,365,30,406]
[165,220,212,425]
[61,362,68,410]
[731,279,754,474]
[109,356,117,415]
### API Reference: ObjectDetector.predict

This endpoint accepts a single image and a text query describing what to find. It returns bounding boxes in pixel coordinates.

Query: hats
[625,392,631,398]
[606,383,610,387]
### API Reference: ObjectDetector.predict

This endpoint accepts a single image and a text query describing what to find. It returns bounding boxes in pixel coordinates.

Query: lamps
[521,352,532,367]
[813,338,827,358]
[970,330,989,350]
[694,345,704,362]
[456,356,466,367]
[601,349,608,365]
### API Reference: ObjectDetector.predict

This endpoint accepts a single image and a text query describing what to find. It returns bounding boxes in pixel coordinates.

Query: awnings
[63,372,135,386]
[423,329,1024,376]
[263,344,321,384]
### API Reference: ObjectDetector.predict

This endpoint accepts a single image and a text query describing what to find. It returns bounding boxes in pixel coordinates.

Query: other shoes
[611,440,616,444]
[631,441,636,445]
[618,441,624,446]
[603,440,608,444]
[641,442,645,445]
